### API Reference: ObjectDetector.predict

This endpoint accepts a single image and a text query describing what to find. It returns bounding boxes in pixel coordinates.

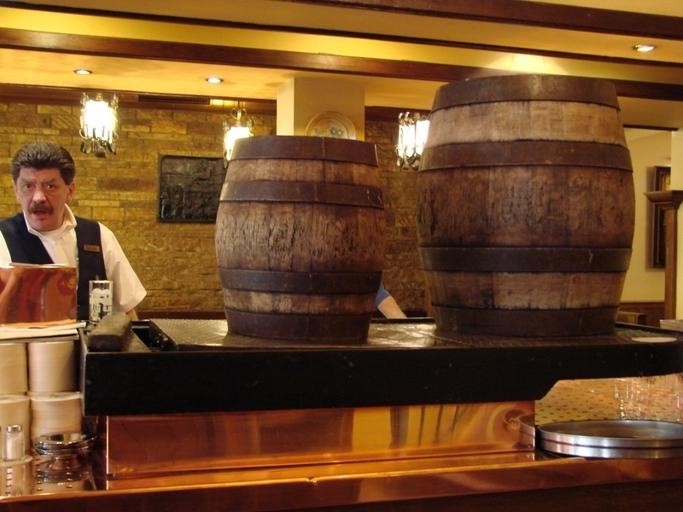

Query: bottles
[4,424,25,494]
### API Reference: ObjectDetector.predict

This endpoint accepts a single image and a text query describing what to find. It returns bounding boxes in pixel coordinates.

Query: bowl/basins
[35,432,95,467]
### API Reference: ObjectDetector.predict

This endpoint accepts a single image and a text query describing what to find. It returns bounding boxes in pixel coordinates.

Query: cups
[88,279,113,326]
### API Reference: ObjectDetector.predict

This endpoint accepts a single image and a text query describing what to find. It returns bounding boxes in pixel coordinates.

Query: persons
[1,144,149,321]
[375,283,405,318]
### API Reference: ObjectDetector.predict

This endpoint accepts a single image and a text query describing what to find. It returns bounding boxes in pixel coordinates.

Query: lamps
[221,99,256,172]
[394,110,432,172]
[77,90,123,160]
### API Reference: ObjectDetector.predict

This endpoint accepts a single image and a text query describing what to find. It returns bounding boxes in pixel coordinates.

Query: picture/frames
[646,165,672,273]
[158,154,232,225]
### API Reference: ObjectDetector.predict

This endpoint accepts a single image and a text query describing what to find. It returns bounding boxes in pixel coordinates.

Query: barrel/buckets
[413,76,636,336]
[213,134,385,345]
